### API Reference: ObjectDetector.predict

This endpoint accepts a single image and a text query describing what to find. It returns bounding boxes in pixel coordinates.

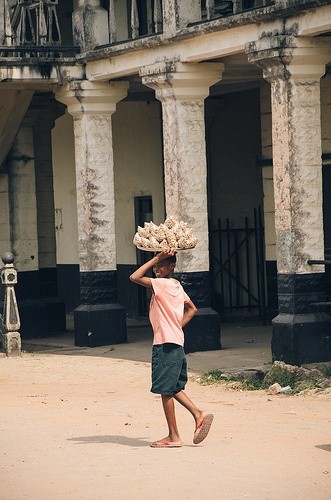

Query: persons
[129,247,214,448]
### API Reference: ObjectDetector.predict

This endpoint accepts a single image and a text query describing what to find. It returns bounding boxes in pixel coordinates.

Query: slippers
[150,440,182,448]
[193,414,214,444]
